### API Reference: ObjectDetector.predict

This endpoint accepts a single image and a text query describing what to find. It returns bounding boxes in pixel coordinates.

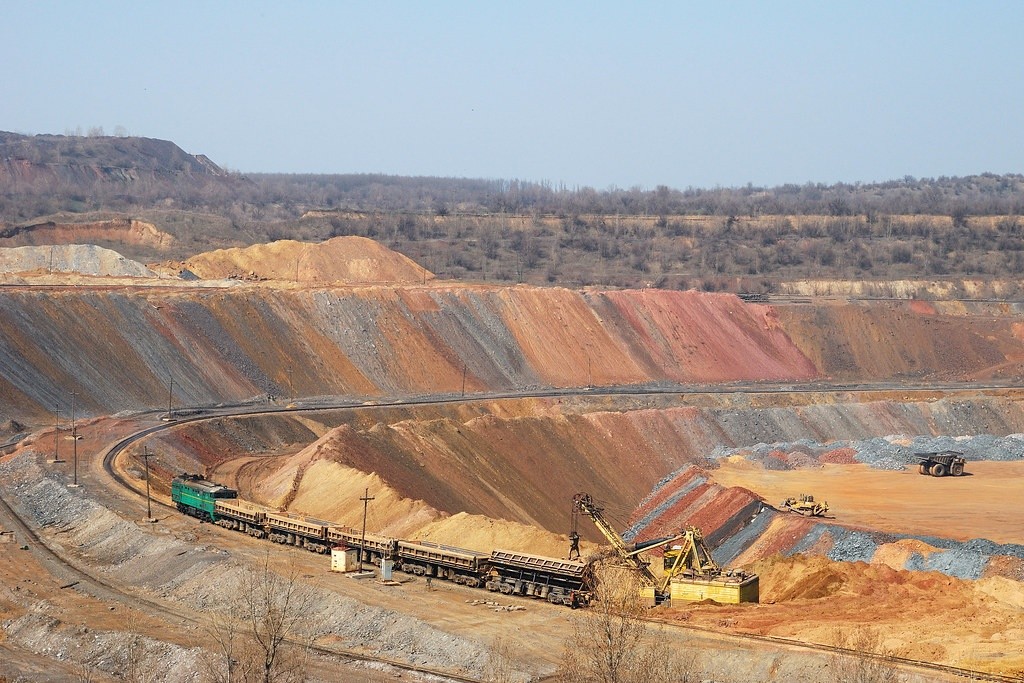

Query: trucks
[913,449,968,477]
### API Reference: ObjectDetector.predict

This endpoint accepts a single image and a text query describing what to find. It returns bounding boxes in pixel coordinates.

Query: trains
[170,472,598,612]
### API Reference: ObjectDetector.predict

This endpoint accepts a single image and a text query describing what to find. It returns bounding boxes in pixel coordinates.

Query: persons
[569,532,581,560]
[426,576,434,592]
[570,589,578,610]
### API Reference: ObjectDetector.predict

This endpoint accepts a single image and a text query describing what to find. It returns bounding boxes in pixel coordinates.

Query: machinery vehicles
[778,493,831,517]
[568,490,760,608]
